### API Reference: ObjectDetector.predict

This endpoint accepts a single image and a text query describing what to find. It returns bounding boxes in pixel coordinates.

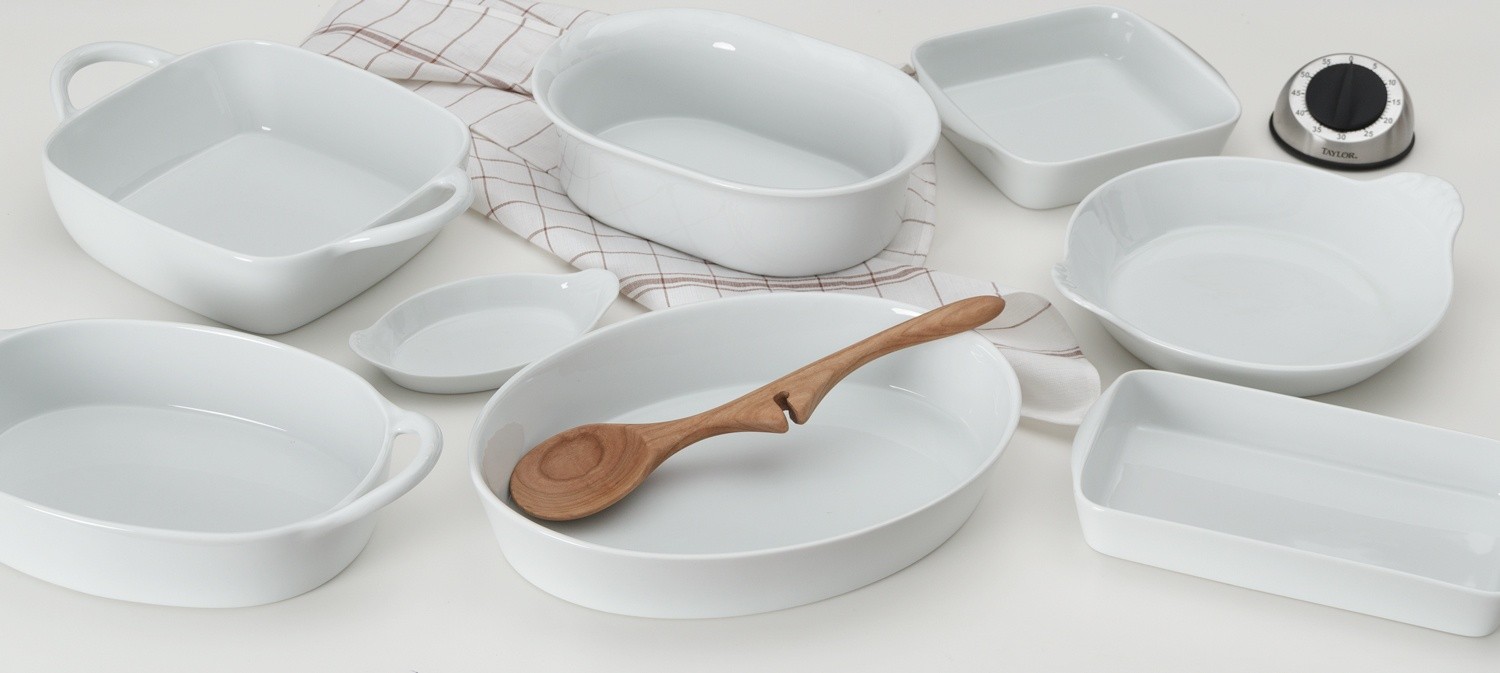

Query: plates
[347,262,621,395]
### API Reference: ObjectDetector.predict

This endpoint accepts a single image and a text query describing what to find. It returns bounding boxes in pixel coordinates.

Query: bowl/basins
[910,5,1242,212]
[38,37,473,335]
[1050,155,1464,398]
[532,15,945,276]
[1068,368,1500,642]
[0,315,444,615]
[465,293,1023,618]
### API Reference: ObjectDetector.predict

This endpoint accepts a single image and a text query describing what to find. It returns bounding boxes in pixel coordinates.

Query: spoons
[508,294,1009,524]
